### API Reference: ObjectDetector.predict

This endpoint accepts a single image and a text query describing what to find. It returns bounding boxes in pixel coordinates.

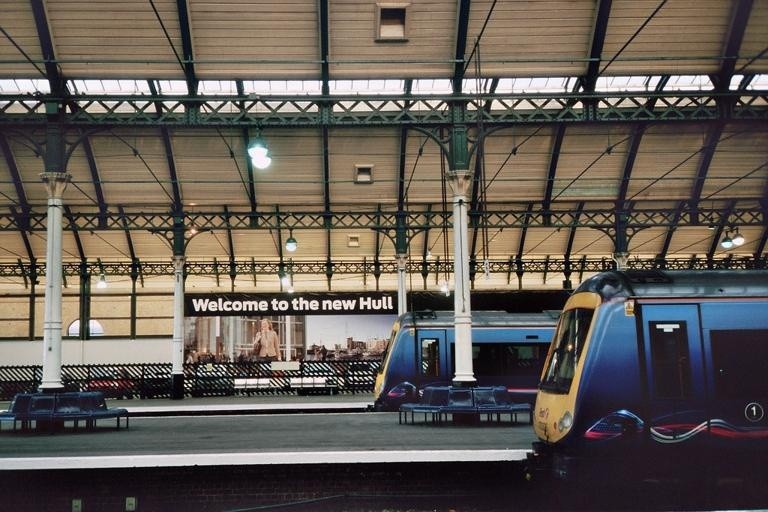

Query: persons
[185,344,253,362]
[311,342,361,362]
[254,318,281,361]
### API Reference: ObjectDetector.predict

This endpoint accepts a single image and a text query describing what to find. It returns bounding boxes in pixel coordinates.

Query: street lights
[356,345,359,364]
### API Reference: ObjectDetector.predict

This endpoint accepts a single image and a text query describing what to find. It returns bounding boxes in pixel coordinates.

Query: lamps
[247,100,271,169]
[286,228,297,251]
[720,228,744,248]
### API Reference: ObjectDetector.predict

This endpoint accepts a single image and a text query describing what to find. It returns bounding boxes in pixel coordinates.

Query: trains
[520,270,768,506]
[363,310,562,411]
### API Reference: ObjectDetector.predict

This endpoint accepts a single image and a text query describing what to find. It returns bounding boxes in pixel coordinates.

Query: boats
[366,351,381,356]
[334,347,362,360]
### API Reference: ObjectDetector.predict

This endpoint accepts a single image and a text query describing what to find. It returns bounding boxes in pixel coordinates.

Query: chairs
[0,392,128,434]
[398,384,533,426]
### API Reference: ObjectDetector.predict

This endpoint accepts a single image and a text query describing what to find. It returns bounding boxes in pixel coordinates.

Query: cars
[288,362,339,396]
[342,360,382,391]
[190,363,234,397]
[27,371,80,399]
[82,367,133,401]
[139,370,173,398]
